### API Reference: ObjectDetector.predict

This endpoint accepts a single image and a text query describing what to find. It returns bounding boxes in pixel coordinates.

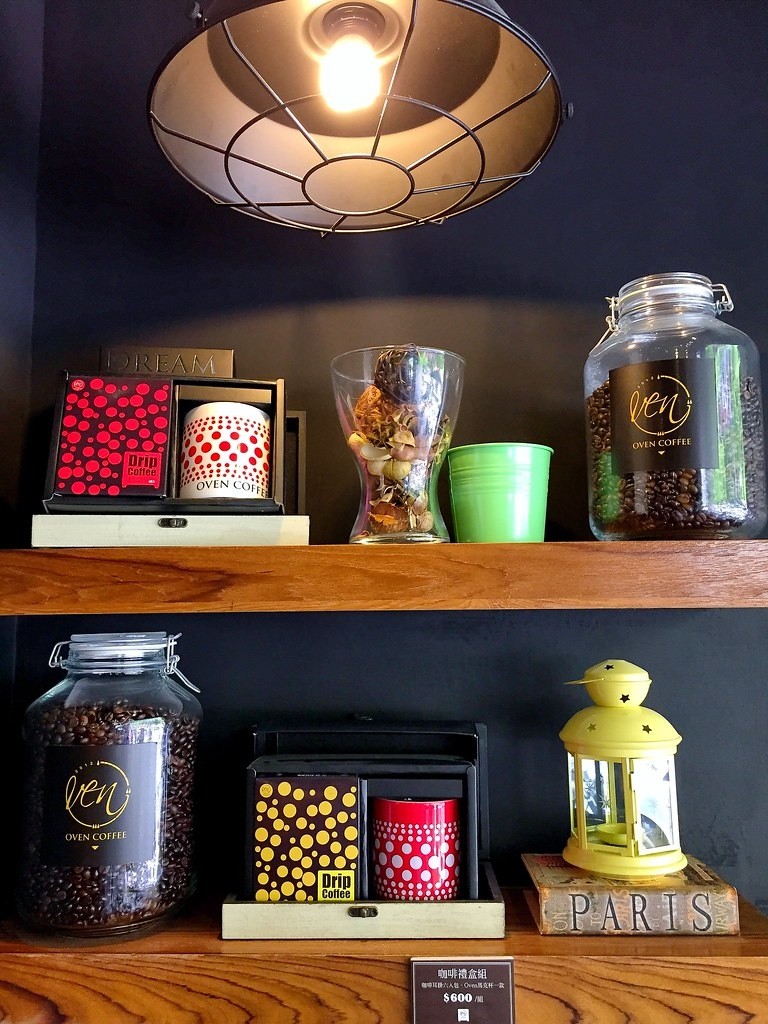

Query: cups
[445,441,555,544]
[369,797,461,903]
[178,401,271,502]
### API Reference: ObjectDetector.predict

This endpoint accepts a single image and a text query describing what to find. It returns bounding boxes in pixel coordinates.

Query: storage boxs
[29,369,311,545]
[219,709,509,943]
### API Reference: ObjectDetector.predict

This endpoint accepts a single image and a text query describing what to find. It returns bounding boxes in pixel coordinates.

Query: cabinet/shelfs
[0,532,767,1024]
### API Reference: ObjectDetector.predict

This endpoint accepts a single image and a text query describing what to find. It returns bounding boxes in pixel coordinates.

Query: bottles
[584,272,768,541]
[16,630,206,942]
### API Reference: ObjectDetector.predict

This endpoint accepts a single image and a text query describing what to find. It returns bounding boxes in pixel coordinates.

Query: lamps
[149,1,568,233]
